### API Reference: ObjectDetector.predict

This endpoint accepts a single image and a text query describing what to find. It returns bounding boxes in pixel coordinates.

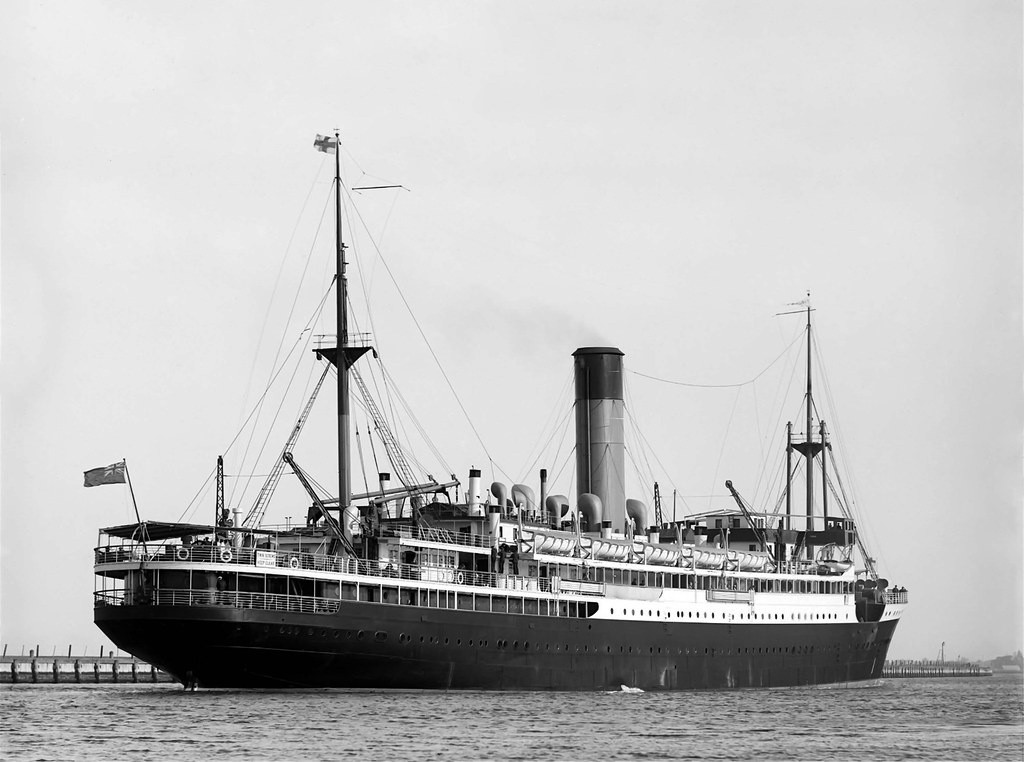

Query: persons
[216,576,226,605]
[631,578,645,586]
[892,585,908,604]
[201,537,232,563]
[725,583,817,594]
[688,581,693,589]
[406,599,412,605]
[457,564,467,582]
[837,523,841,529]
[829,523,832,529]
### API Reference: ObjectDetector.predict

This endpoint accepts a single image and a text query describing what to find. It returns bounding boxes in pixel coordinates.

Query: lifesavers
[220,550,233,562]
[458,572,463,584]
[290,557,299,569]
[177,549,189,560]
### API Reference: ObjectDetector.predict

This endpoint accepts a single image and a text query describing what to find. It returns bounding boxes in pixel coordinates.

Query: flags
[84,462,126,488]
[313,134,337,155]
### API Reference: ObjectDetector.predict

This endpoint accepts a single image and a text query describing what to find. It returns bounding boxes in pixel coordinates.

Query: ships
[88,121,909,696]
